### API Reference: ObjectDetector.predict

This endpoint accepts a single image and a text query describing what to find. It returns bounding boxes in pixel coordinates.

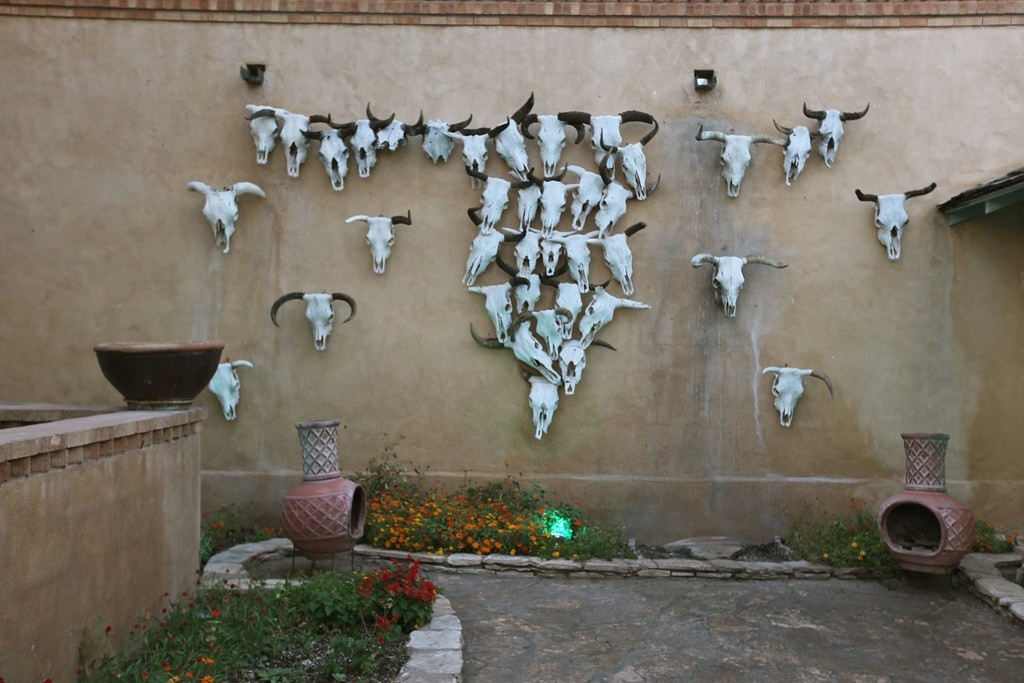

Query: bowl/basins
[93,341,225,411]
